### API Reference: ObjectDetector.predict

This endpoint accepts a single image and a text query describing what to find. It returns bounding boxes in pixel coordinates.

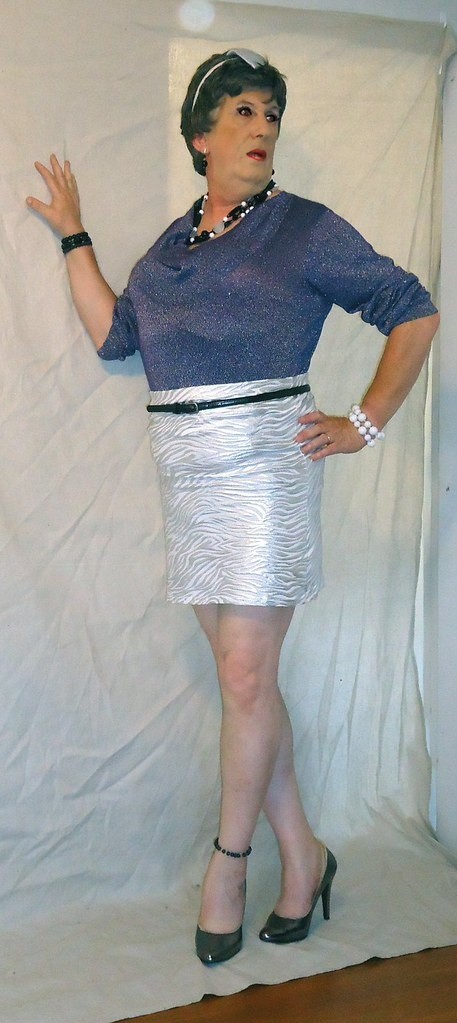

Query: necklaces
[188,179,277,244]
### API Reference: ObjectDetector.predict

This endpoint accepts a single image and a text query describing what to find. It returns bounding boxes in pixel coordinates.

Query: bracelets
[61,232,92,253]
[350,404,385,448]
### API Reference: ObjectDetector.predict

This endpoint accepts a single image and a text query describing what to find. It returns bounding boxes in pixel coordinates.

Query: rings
[326,433,331,443]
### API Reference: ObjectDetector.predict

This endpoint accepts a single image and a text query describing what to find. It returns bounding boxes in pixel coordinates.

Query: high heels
[195,880,247,963]
[258,845,337,945]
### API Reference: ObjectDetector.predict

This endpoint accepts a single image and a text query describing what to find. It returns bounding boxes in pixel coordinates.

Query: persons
[27,47,439,964]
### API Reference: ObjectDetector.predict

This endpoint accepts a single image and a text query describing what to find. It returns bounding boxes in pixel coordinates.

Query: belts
[145,385,314,412]
